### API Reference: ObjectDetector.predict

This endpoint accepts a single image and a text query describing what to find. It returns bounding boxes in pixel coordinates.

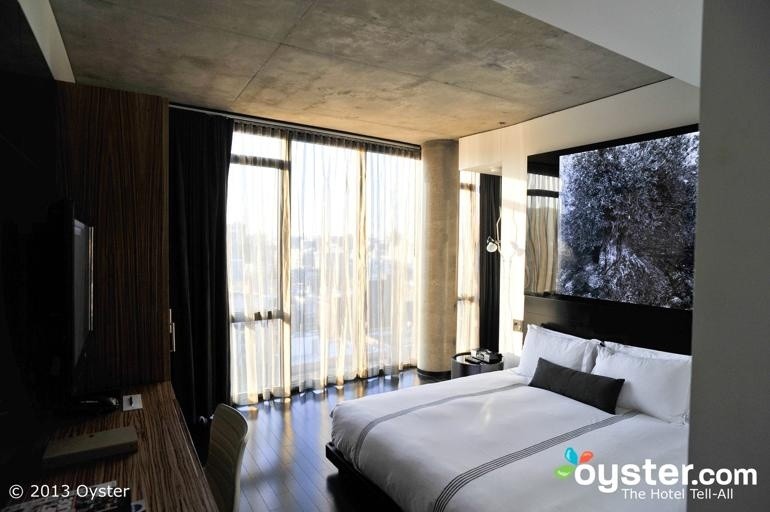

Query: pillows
[591,340,692,426]
[519,325,598,374]
[528,358,625,414]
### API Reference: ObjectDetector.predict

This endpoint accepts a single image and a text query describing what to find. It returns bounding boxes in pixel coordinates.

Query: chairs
[202,403,249,512]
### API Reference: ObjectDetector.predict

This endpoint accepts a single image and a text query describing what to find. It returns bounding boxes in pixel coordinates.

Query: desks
[0,379,218,510]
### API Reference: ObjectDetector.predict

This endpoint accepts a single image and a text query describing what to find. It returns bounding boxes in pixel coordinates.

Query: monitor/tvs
[24,199,119,421]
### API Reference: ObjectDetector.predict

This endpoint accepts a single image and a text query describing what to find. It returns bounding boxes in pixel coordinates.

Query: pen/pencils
[129,396,134,407]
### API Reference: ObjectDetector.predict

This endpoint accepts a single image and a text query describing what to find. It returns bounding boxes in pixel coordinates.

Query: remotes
[466,356,482,364]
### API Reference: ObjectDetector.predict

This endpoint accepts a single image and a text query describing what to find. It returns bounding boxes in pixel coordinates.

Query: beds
[327,291,693,511]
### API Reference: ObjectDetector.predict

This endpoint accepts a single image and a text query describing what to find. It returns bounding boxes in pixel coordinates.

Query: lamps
[485,216,501,255]
